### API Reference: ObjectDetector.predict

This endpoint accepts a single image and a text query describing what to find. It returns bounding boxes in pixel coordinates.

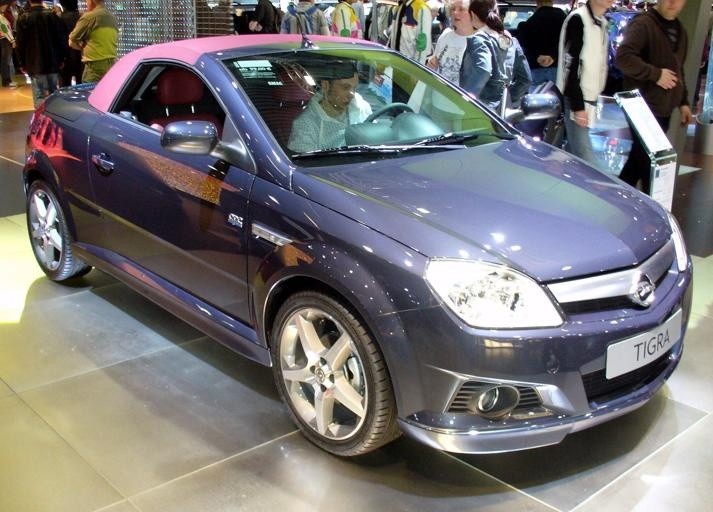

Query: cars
[22,8,693,462]
[604,11,646,83]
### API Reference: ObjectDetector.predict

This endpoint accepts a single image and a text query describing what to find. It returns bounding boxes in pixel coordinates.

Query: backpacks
[287,5,317,34]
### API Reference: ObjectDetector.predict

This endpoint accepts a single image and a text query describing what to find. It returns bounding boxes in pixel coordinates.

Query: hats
[59,0,77,11]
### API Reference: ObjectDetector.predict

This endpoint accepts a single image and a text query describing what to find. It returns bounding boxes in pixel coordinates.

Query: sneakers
[9,82,17,86]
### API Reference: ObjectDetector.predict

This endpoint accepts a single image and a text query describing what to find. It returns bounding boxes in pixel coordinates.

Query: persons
[232,1,692,197]
[0,1,121,114]
